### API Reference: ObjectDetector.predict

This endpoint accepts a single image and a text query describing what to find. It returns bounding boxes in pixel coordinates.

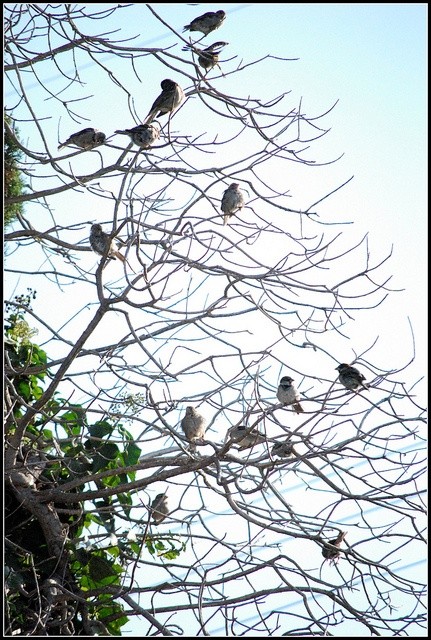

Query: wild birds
[322,531,348,560]
[143,79,184,124]
[181,405,205,455]
[150,493,169,522]
[114,124,160,151]
[334,363,370,394]
[220,183,243,227]
[226,426,267,448]
[275,376,304,415]
[182,9,225,36]
[88,224,124,261]
[270,441,292,457]
[181,41,229,68]
[56,128,105,150]
